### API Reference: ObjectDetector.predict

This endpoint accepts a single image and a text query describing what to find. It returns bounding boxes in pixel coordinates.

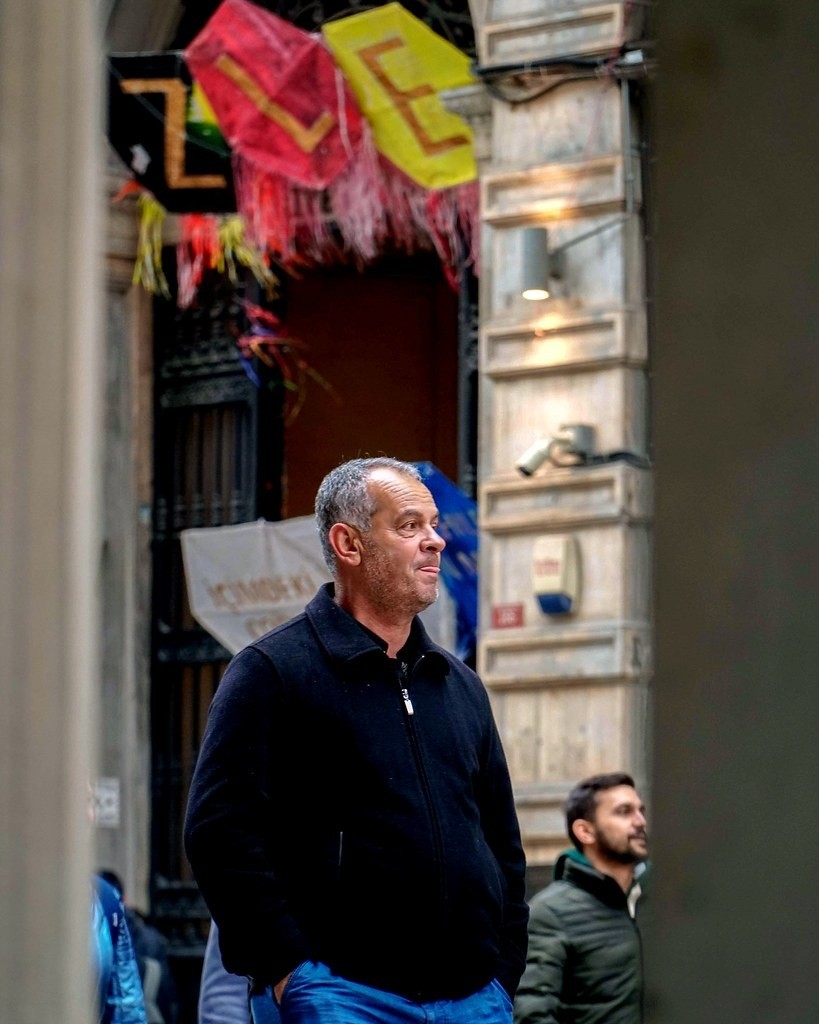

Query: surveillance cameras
[516,439,555,477]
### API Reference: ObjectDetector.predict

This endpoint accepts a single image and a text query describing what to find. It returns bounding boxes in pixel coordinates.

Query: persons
[520,772,660,1024]
[179,456,529,1024]
[91,866,172,1024]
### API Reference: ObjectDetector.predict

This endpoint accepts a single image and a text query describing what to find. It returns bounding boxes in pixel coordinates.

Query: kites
[105,0,483,401]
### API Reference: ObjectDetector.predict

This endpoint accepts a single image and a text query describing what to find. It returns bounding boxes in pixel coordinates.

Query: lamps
[514,79,633,302]
